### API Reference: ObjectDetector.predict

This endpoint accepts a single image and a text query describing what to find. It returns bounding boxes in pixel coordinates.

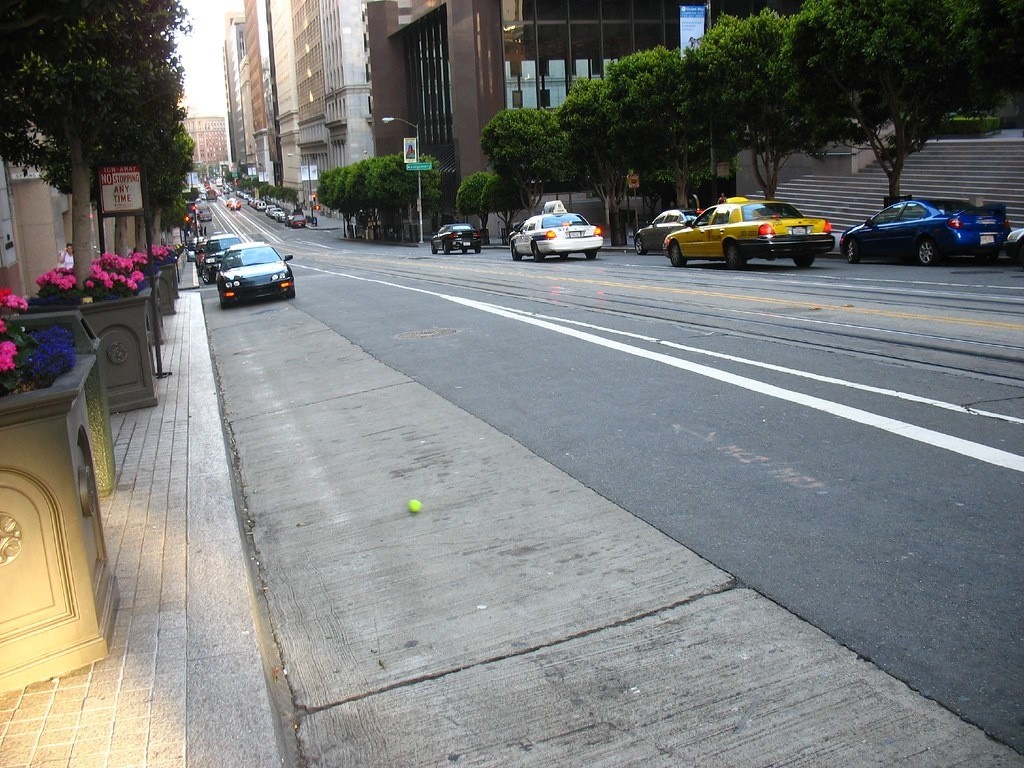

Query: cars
[663,197,835,271]
[197,177,306,228]
[431,223,482,254]
[210,241,296,308]
[634,209,705,255]
[1002,228,1024,263]
[184,233,243,284]
[508,199,604,263]
[839,198,1013,266]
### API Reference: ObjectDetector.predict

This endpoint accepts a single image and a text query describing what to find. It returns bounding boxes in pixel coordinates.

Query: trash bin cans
[5,309,115,498]
[884,195,911,210]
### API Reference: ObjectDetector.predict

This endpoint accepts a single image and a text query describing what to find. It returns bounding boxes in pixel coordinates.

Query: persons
[57,243,74,270]
[717,193,728,204]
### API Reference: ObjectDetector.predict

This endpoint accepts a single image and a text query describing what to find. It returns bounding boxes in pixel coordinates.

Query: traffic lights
[314,205,320,210]
[182,203,196,231]
[313,195,316,201]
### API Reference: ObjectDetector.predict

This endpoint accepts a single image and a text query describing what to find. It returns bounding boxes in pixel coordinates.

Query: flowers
[0,243,185,396]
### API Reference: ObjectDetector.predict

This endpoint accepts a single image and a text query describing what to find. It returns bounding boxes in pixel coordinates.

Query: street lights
[382,117,425,244]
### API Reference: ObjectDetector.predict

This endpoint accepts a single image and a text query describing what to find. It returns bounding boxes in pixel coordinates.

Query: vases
[1,263,177,696]
